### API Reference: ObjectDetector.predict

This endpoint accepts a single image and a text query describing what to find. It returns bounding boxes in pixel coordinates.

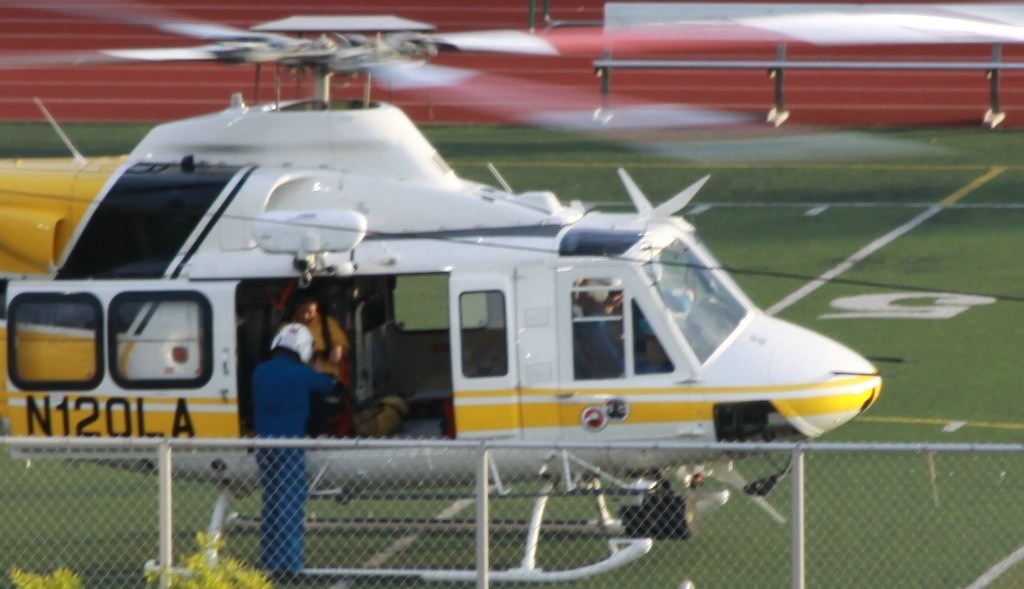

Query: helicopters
[1,14,885,587]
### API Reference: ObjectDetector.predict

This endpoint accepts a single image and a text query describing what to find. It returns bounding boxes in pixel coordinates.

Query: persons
[578,277,693,373]
[249,323,337,586]
[279,294,351,436]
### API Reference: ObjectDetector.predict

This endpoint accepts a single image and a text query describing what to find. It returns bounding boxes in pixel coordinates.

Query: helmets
[271,323,314,364]
[580,274,623,303]
